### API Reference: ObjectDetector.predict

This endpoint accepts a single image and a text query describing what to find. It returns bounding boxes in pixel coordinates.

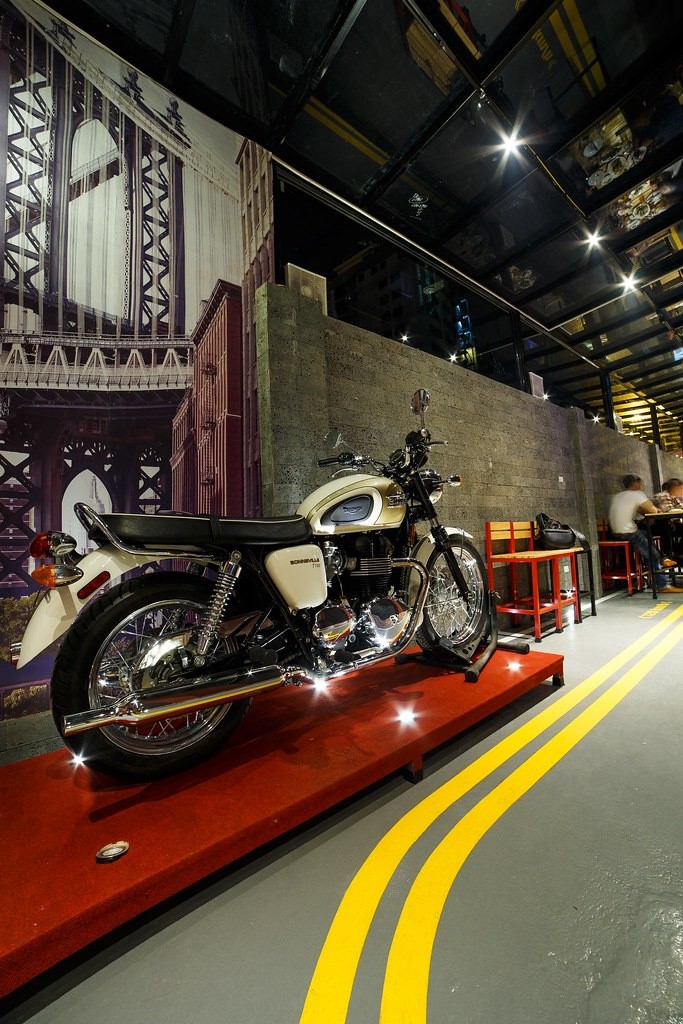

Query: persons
[637,478,683,567]
[607,474,683,592]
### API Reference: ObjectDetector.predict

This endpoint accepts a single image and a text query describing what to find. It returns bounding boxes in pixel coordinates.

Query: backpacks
[536,511,591,552]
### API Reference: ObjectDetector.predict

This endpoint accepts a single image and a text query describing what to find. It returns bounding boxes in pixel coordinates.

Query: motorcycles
[17,388,489,784]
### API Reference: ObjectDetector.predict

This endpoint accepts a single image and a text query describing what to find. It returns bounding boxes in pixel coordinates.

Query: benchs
[486,520,597,643]
[596,519,660,597]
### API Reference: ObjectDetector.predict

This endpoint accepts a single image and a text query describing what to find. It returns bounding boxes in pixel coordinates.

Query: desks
[645,511,683,599]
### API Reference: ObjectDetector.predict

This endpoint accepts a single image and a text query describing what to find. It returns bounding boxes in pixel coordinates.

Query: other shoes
[663,559,677,567]
[660,585,683,593]
